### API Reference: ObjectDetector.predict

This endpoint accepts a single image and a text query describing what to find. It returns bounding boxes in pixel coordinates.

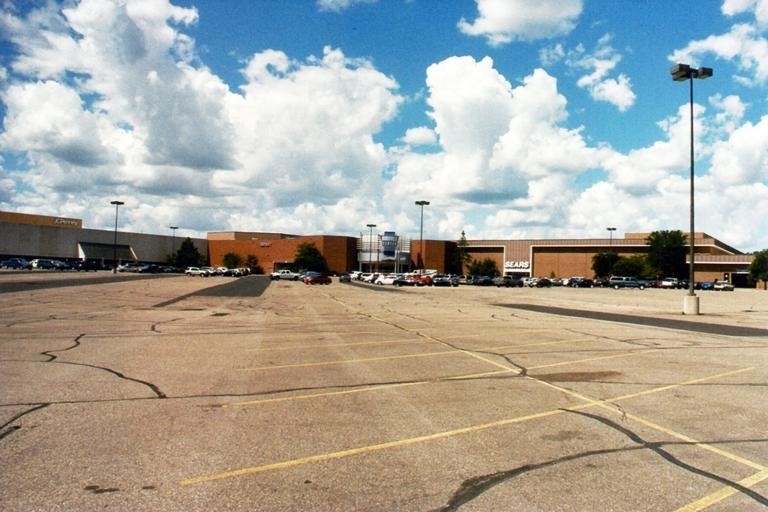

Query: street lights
[415,200,430,276]
[606,228,616,244]
[170,226,178,257]
[110,201,125,275]
[671,64,713,318]
[367,224,376,273]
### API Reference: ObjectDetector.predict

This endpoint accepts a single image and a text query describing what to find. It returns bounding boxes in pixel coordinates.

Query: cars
[0,258,252,277]
[270,269,332,285]
